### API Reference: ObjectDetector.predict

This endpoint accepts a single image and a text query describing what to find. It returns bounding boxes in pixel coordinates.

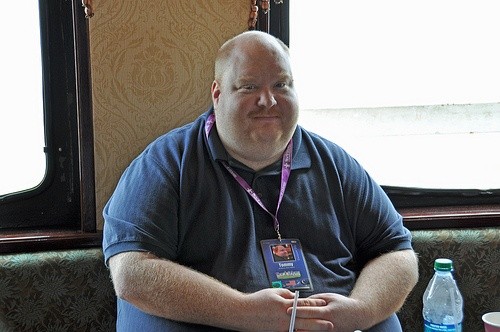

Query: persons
[102,31,418,332]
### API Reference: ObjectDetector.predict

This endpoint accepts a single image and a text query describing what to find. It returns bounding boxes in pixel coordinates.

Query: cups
[481,312,500,332]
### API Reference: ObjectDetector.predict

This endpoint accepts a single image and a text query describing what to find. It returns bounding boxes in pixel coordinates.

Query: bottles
[422,258,463,332]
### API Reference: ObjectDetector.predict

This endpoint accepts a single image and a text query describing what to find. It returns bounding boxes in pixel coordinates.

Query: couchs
[0,205,500,332]
